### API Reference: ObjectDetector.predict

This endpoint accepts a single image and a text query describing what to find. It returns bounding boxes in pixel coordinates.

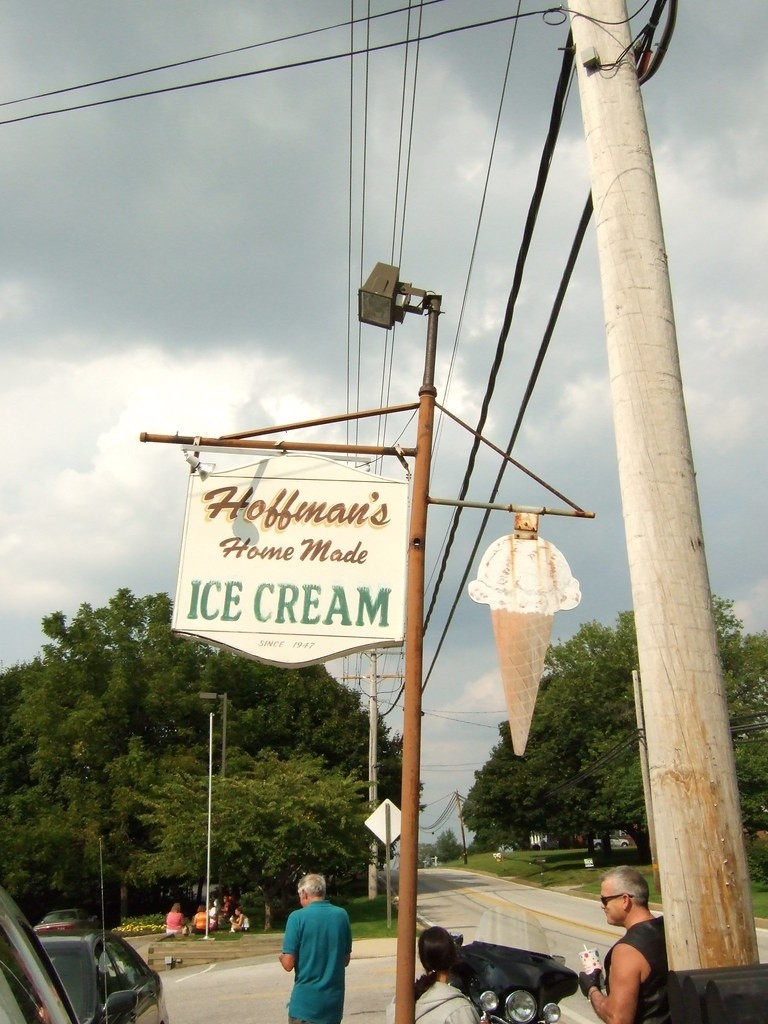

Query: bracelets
[589,989,597,1003]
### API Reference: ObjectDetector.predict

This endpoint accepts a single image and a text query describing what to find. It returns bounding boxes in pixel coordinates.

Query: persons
[578,866,669,1024]
[278,874,352,1024]
[208,900,218,931]
[221,895,240,921]
[386,926,481,1024]
[229,907,250,932]
[166,903,185,934]
[191,905,210,934]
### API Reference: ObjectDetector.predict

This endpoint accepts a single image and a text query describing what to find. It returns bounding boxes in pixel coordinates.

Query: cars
[593,834,630,849]
[36,927,170,1024]
[38,908,103,935]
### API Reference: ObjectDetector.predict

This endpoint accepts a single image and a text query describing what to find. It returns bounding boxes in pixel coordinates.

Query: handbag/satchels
[182,925,189,935]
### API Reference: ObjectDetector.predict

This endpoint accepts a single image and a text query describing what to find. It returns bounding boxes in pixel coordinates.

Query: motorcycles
[422,927,578,1024]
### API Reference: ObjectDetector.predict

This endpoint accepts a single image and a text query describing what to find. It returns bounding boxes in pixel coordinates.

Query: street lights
[195,689,227,776]
[357,261,445,1024]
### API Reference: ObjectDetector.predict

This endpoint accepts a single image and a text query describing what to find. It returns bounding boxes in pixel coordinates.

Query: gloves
[577,968,602,997]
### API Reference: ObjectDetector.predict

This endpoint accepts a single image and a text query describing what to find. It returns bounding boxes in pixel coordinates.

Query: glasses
[601,894,633,905]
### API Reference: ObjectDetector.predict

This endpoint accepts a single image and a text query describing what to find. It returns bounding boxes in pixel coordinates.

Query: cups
[578,948,607,990]
